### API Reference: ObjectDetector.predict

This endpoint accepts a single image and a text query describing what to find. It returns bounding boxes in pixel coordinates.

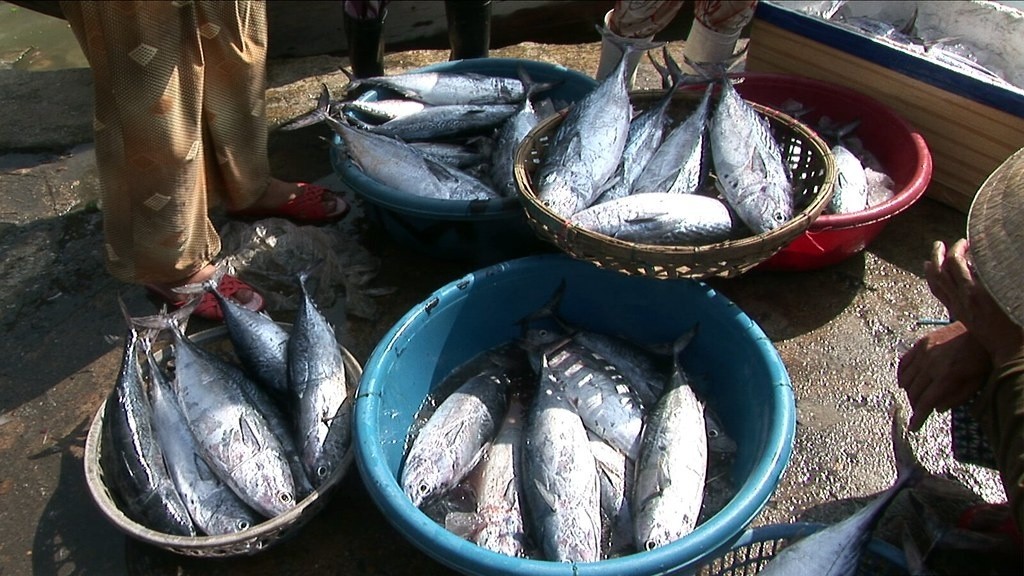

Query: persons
[595,0,760,93]
[897,147,1024,556]
[56,0,351,320]
[343,0,492,78]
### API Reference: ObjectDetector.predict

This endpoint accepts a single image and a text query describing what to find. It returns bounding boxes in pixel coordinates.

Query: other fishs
[400,274,739,563]
[534,24,897,247]
[276,64,574,202]
[105,256,353,537]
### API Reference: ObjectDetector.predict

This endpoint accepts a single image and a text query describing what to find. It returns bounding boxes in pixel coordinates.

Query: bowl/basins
[327,58,596,264]
[675,70,932,275]
[353,250,797,576]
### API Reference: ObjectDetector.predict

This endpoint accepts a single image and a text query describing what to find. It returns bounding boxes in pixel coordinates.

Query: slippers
[163,266,266,325]
[262,177,347,225]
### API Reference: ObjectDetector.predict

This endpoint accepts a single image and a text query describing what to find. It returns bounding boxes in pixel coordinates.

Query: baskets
[513,81,837,280]
[80,317,371,556]
